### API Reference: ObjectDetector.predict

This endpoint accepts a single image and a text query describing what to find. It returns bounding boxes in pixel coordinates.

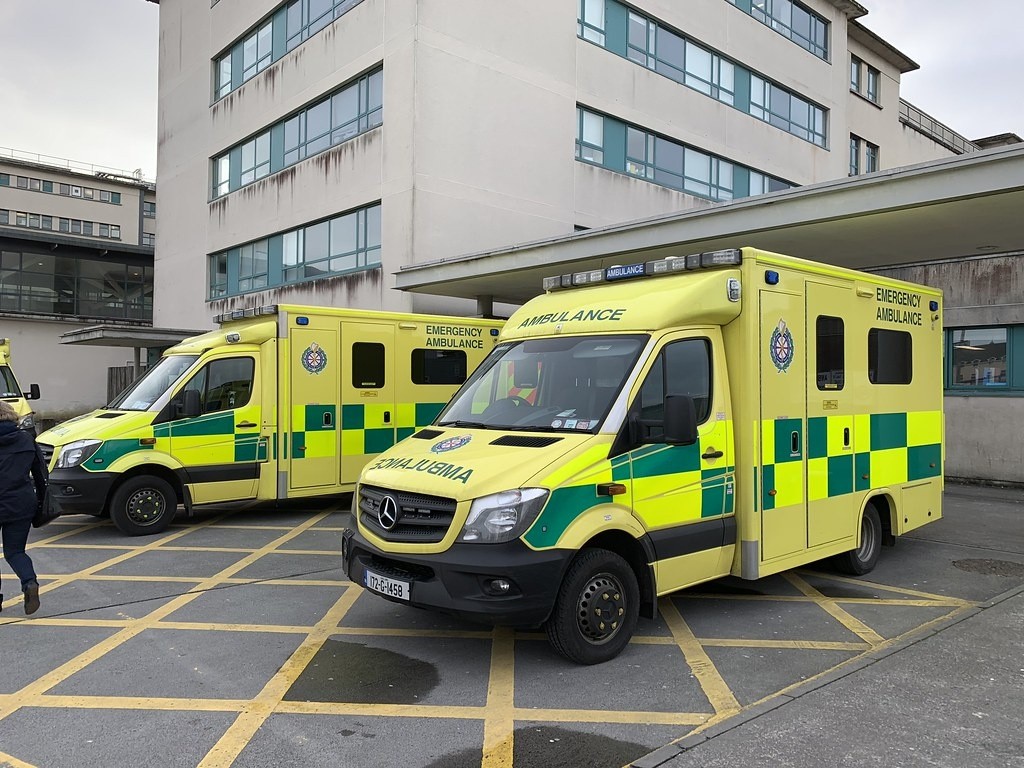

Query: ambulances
[34,302,508,536]
[1,337,41,448]
[341,246,946,666]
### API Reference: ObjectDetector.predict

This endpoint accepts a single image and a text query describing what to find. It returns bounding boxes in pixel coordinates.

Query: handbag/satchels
[30,484,62,529]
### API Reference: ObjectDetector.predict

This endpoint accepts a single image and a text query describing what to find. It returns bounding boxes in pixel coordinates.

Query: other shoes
[22,579,40,615]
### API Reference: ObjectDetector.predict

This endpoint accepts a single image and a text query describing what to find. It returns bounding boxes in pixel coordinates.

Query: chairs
[642,358,694,438]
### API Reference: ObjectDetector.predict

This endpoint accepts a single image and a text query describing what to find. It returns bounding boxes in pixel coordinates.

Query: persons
[0,401,49,614]
